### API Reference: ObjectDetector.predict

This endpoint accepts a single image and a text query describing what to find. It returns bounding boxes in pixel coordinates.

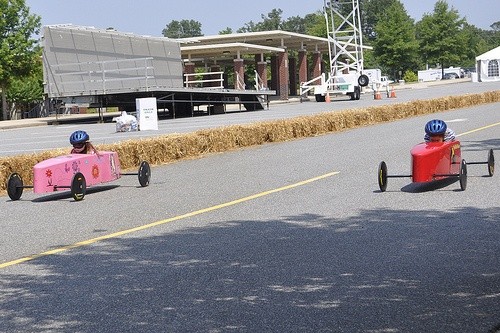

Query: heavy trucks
[417,67,471,82]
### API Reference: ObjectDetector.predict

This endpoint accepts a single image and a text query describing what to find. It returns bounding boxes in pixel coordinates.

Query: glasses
[73,143,86,148]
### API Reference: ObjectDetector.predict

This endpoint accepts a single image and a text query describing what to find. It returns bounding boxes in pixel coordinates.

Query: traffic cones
[325,89,331,102]
[376,88,381,100]
[389,85,397,99]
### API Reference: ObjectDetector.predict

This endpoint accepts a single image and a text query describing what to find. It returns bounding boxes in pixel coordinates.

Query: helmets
[70,130,90,144]
[425,119,447,135]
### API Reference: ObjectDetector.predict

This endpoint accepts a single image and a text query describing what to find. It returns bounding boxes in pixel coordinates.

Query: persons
[424,120,455,143]
[69,130,95,154]
